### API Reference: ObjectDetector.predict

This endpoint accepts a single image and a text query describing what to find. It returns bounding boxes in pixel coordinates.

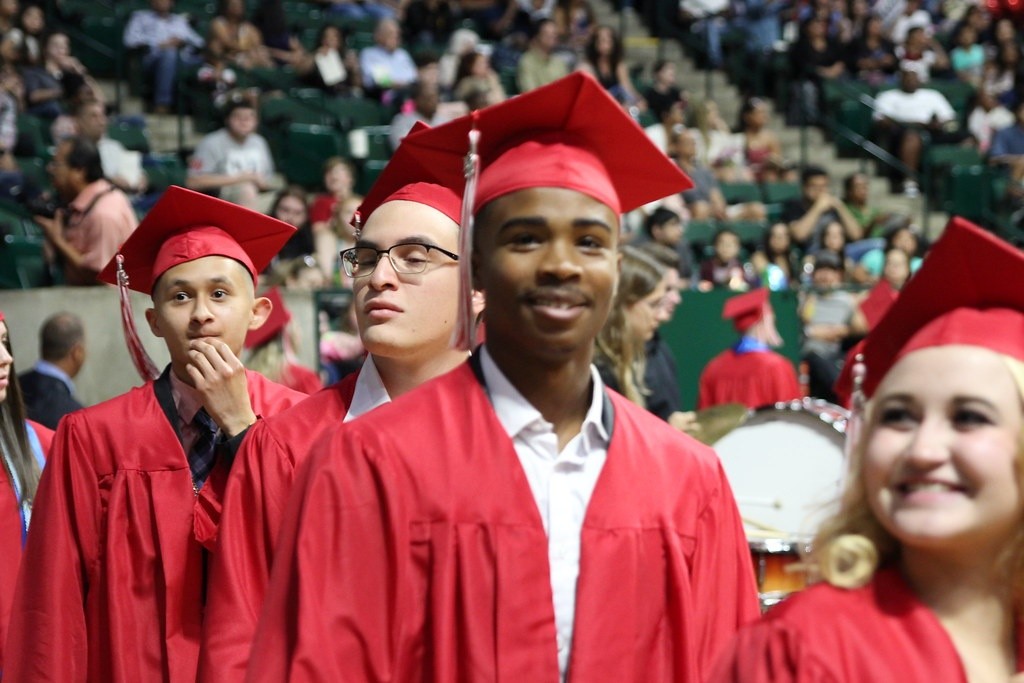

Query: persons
[0,185,312,683]
[0,0,1024,425]
[194,114,487,683]
[244,70,761,683]
[702,216,1024,683]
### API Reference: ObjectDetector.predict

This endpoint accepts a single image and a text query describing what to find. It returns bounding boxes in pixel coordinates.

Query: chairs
[0,1,1024,413]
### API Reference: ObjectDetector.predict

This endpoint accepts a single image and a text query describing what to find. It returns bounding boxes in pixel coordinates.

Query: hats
[94,184,298,382]
[351,119,462,230]
[401,70,697,353]
[862,216,1024,399]
[858,280,898,331]
[722,287,770,331]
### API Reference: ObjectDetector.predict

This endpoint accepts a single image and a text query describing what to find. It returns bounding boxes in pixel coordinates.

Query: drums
[705,396,854,606]
